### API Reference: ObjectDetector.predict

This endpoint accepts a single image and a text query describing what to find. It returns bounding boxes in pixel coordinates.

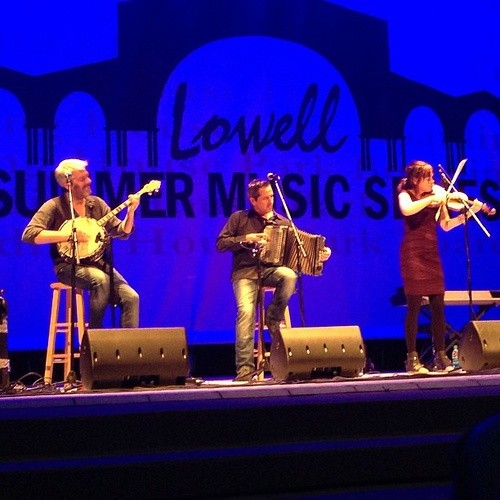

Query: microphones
[267,173,280,180]
[439,164,451,180]
[64,171,72,178]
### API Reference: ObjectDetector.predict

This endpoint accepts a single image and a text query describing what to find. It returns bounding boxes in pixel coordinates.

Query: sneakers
[434,350,455,371]
[405,352,429,374]
[265,319,281,333]
[235,366,253,380]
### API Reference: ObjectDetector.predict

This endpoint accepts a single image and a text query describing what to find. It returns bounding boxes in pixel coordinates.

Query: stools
[44,279,89,383]
[253,285,291,382]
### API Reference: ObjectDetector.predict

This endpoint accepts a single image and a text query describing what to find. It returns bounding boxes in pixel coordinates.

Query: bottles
[452,345,459,368]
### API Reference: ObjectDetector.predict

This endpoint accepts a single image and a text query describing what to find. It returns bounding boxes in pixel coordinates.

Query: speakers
[269,326,366,381]
[459,320,500,371]
[79,328,190,391]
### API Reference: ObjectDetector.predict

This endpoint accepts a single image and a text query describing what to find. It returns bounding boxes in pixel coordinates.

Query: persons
[21,158,140,328]
[216,179,332,382]
[397,160,484,374]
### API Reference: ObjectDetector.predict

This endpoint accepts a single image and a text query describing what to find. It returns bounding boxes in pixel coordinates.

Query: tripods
[233,257,271,382]
[24,177,82,393]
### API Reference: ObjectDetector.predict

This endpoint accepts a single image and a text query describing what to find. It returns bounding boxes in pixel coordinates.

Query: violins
[421,184,496,215]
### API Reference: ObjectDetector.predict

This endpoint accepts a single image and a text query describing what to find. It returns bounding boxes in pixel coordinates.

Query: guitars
[56,180,161,263]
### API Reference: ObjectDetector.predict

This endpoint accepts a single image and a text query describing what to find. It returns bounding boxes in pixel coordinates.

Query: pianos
[391,285,500,307]
